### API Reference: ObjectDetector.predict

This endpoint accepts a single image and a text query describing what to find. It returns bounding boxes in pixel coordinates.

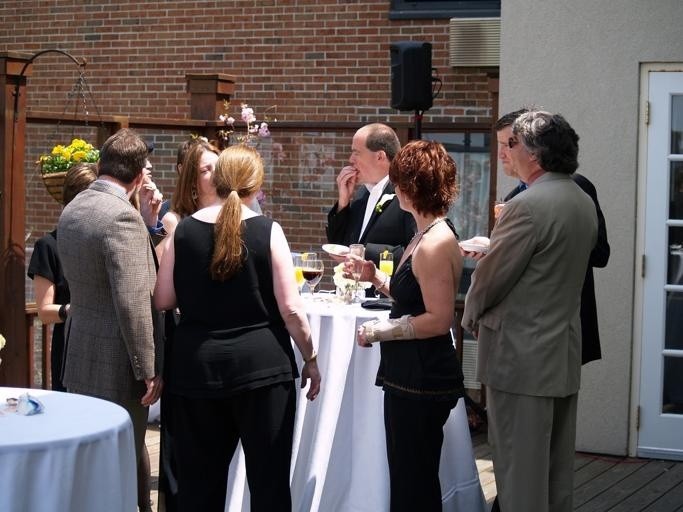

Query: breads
[462,237,490,247]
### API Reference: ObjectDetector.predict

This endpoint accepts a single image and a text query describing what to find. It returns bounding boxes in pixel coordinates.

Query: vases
[42,175,67,204]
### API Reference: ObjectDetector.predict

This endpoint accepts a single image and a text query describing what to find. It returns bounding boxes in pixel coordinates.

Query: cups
[494,202,506,220]
[378,252,393,276]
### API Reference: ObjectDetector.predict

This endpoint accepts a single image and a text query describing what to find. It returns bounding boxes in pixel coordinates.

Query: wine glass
[291,252,324,299]
[346,244,365,303]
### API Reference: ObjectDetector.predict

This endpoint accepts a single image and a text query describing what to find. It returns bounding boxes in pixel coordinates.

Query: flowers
[186,98,287,207]
[36,139,102,173]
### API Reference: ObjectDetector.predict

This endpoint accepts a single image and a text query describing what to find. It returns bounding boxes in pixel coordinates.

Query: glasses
[507,137,523,148]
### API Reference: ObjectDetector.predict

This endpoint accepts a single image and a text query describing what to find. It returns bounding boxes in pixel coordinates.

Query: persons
[23,129,165,424]
[122,158,176,512]
[325,122,416,300]
[356,138,467,510]
[154,141,323,511]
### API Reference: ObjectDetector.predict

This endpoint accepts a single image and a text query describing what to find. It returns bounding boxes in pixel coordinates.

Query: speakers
[389,41,433,111]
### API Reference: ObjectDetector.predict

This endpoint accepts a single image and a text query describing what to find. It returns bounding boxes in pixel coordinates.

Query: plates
[456,242,487,255]
[321,244,350,256]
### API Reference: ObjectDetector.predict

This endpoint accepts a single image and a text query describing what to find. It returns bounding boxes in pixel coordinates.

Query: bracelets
[376,273,387,291]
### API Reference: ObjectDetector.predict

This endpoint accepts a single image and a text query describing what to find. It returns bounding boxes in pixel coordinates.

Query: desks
[290,290,457,512]
[0,386,135,510]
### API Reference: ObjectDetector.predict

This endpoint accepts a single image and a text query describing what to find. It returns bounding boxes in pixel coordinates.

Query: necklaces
[408,219,444,243]
